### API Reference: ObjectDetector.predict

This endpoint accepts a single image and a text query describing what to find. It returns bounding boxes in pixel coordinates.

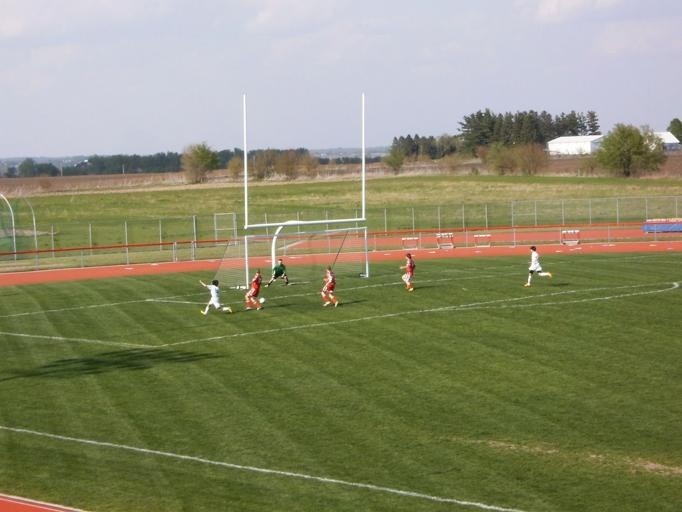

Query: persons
[198,279,233,316]
[264,259,289,288]
[243,268,265,311]
[524,245,552,287]
[319,264,339,308]
[399,253,416,292]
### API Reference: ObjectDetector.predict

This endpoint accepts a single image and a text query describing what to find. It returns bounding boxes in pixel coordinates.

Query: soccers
[259,297,265,303]
